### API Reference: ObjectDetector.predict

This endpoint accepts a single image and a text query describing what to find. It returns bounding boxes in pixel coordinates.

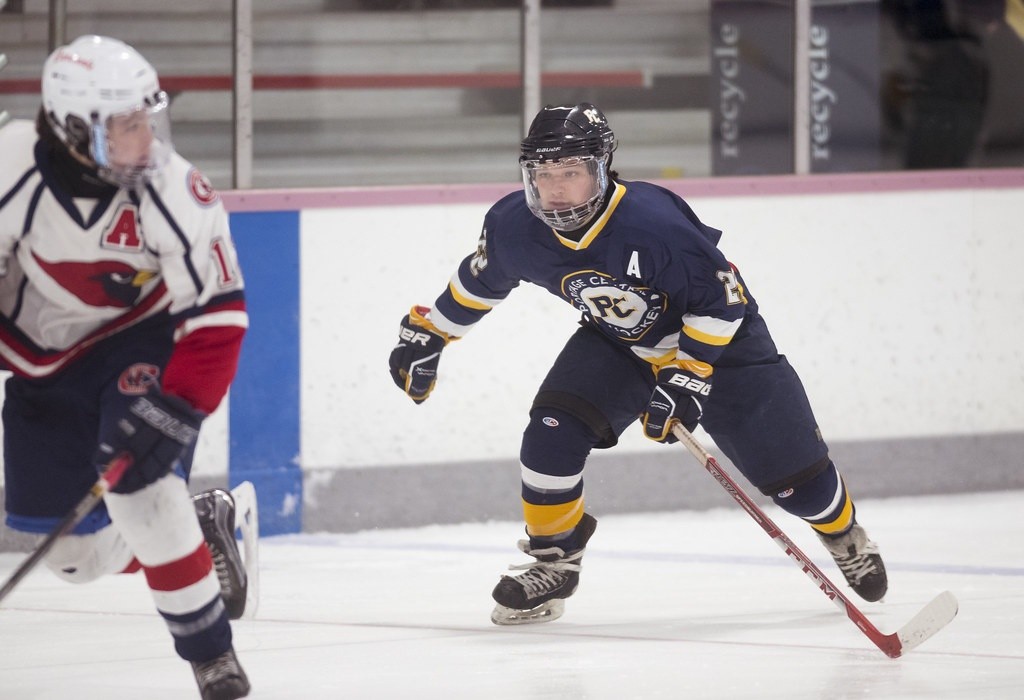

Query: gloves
[389,305,460,404]
[92,386,206,495]
[640,358,716,445]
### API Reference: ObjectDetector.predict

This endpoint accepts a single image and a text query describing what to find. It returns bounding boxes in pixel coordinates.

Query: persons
[388,102,887,626]
[0,34,258,700]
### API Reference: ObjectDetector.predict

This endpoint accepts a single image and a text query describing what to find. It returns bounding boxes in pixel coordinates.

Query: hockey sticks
[669,419,962,659]
[0,448,138,602]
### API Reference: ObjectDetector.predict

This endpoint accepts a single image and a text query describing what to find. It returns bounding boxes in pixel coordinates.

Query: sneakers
[492,511,597,626]
[812,504,888,602]
[189,644,250,700]
[189,481,259,621]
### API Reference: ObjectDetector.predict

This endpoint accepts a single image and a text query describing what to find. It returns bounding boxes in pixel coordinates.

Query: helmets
[41,34,161,155]
[521,102,614,199]
